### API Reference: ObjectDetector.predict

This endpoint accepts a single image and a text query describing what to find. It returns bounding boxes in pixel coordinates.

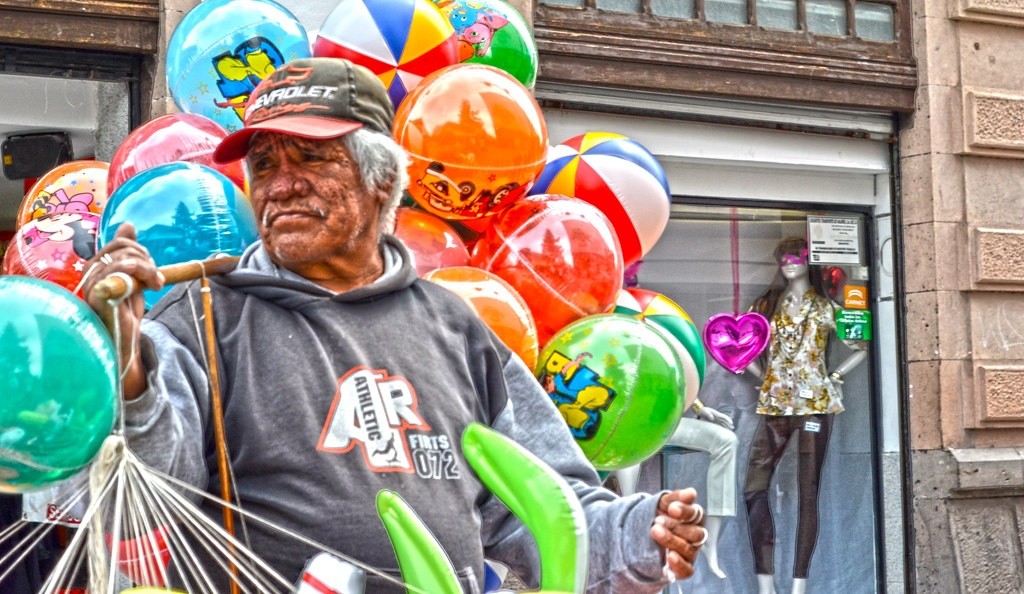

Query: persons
[744,237,868,594]
[81,58,709,594]
[613,397,739,580]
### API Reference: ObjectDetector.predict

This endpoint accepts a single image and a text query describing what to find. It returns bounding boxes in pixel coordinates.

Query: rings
[685,504,703,524]
[103,253,112,263]
[100,256,109,265]
[691,527,708,547]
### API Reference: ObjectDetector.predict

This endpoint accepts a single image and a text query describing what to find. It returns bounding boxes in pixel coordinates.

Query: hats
[213,57,395,166]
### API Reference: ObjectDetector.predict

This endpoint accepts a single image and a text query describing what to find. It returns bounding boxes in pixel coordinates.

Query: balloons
[0,1,705,496]
